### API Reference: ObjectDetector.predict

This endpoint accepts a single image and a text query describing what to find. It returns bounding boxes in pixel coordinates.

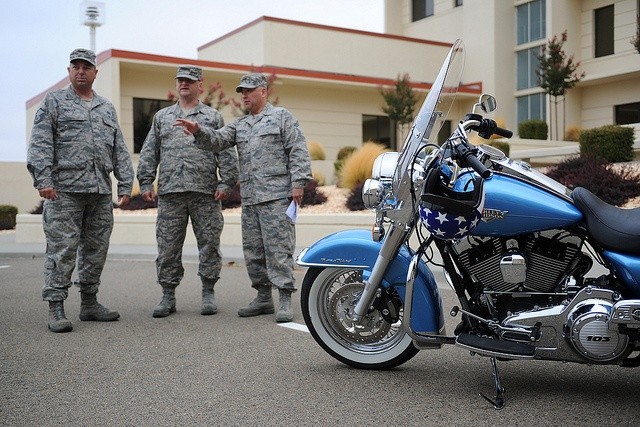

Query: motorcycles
[296,40,637,410]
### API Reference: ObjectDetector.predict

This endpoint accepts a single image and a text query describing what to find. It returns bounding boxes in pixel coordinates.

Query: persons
[136,64,239,318]
[172,72,314,322]
[26,48,134,332]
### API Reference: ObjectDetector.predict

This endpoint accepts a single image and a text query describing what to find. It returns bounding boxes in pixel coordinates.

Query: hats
[174,65,203,81]
[236,74,267,93]
[70,49,96,66]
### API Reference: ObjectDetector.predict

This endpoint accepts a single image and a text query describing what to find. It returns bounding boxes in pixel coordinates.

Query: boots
[152,287,176,317]
[201,286,217,315]
[238,288,274,316]
[48,300,72,333]
[79,293,120,321]
[276,288,294,321]
